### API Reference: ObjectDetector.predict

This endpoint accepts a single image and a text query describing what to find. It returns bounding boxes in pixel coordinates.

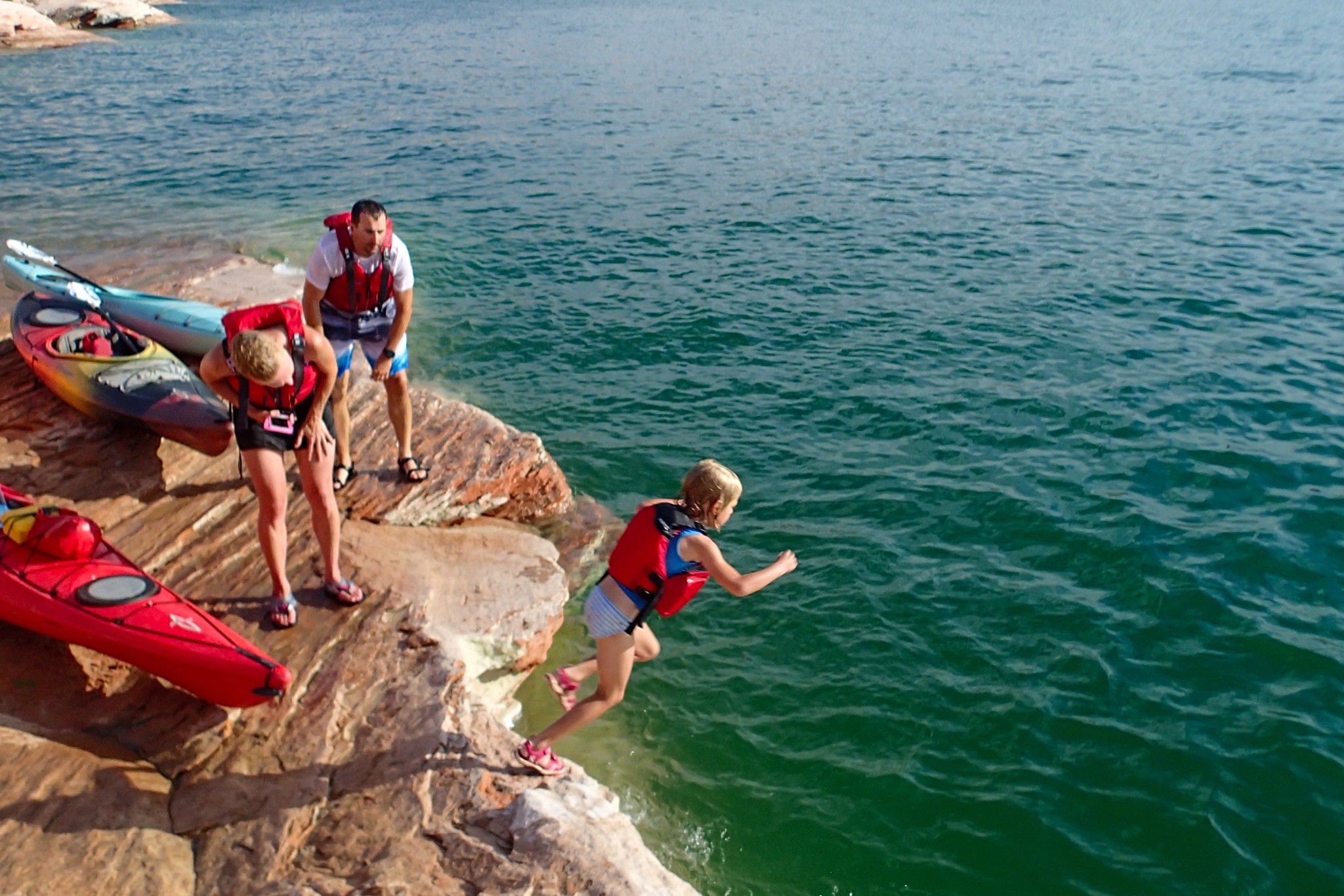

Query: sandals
[332,461,358,491]
[543,668,580,714]
[514,738,566,777]
[397,455,429,483]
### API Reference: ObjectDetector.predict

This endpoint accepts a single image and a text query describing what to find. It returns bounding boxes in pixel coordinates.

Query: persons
[199,301,367,629]
[514,458,798,776]
[302,199,429,493]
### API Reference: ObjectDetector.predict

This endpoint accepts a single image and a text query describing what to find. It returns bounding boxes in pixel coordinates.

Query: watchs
[383,347,395,358]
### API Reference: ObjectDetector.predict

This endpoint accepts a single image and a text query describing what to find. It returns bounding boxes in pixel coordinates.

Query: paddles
[66,282,140,354]
[5,239,114,295]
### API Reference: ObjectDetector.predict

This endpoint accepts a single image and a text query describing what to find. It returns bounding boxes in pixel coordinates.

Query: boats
[0,483,293,709]
[1,254,231,360]
[9,291,236,457]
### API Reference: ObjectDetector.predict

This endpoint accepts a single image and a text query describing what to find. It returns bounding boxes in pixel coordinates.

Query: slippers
[324,576,366,605]
[269,591,298,629]
